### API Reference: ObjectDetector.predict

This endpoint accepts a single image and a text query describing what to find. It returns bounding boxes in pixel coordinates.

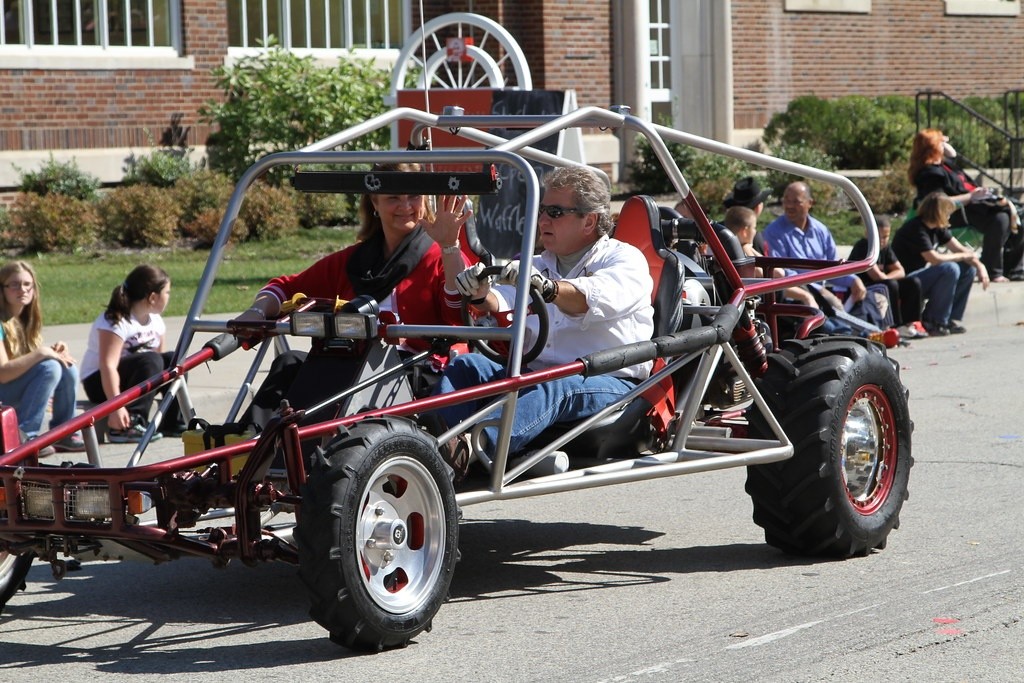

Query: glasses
[539,204,582,219]
[2,280,35,293]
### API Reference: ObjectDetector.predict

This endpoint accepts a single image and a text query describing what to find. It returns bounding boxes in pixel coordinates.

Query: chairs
[613,193,681,447]
[905,199,984,253]
[445,196,492,357]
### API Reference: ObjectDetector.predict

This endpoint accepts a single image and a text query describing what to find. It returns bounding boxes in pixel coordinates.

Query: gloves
[496,260,559,303]
[455,262,493,305]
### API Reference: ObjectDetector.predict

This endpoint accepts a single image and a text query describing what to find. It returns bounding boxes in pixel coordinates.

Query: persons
[889,190,990,334]
[904,130,1023,281]
[712,176,867,336]
[80,262,187,442]
[230,149,657,474]
[0,260,87,455]
[847,216,931,339]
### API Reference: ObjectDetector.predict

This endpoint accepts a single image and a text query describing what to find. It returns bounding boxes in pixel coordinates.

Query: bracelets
[441,242,461,255]
[544,280,559,303]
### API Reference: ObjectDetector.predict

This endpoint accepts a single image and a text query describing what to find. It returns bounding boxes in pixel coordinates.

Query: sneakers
[896,325,917,337]
[159,423,188,438]
[108,423,163,443]
[906,320,929,337]
[51,433,86,451]
[28,435,56,457]
[417,412,472,482]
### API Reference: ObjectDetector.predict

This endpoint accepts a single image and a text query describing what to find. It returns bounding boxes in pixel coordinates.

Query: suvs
[0,105,915,652]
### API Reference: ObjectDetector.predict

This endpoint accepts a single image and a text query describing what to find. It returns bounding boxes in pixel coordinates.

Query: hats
[722,176,775,209]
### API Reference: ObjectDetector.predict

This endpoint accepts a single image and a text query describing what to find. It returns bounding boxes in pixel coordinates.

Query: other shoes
[922,320,950,336]
[1003,269,1024,280]
[948,320,966,334]
[991,275,1010,282]
